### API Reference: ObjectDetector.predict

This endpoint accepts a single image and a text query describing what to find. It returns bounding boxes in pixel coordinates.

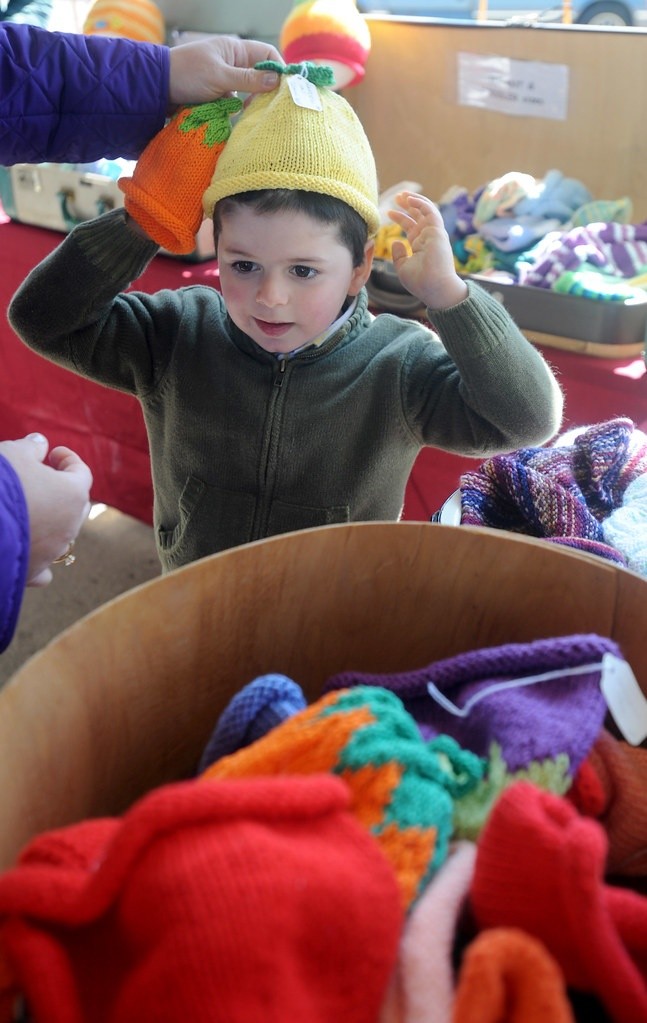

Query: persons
[7,59,564,574]
[0,21,286,657]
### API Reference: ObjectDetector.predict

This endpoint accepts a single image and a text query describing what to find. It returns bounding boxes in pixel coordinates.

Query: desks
[0,219,647,530]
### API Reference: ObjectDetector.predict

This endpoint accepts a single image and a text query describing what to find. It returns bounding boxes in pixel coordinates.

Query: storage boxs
[342,10,647,228]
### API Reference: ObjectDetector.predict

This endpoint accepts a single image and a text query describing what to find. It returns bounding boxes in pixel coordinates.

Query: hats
[279,1,372,89]
[119,95,244,255]
[375,168,647,304]
[2,632,647,1022]
[202,61,380,240]
[83,0,165,47]
